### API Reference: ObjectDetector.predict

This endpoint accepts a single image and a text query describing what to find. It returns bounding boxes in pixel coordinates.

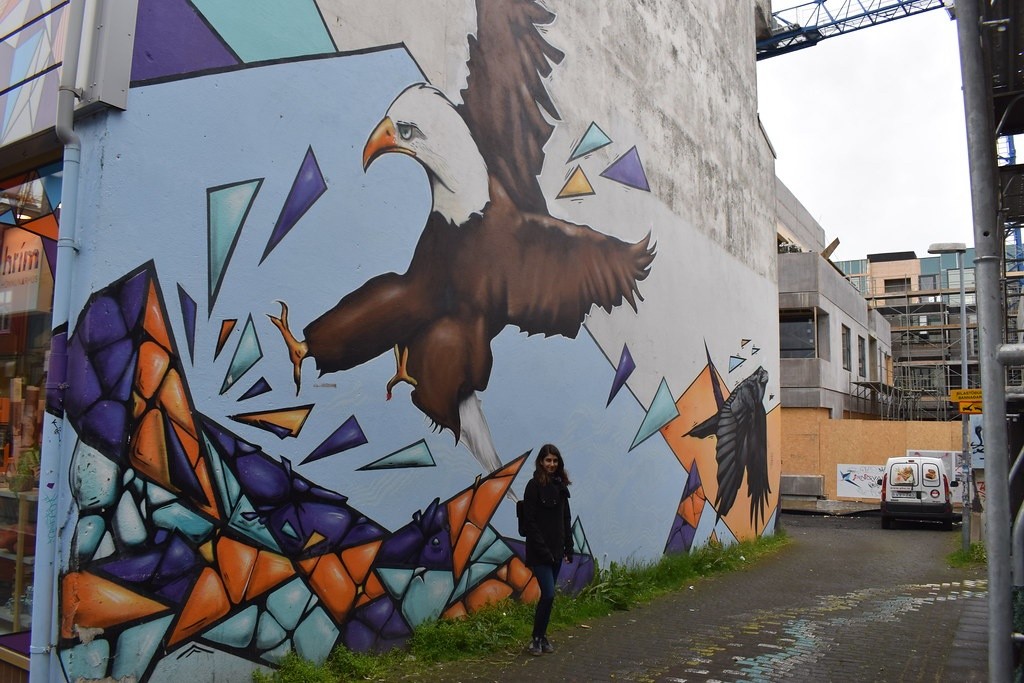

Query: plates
[924,475,938,481]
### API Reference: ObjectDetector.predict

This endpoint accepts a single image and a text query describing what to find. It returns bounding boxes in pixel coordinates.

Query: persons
[521,444,575,655]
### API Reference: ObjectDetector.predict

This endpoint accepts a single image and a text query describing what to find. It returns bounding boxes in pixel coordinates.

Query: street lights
[928,242,971,554]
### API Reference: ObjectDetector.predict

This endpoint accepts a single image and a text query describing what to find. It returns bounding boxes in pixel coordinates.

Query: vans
[876,455,959,530]
